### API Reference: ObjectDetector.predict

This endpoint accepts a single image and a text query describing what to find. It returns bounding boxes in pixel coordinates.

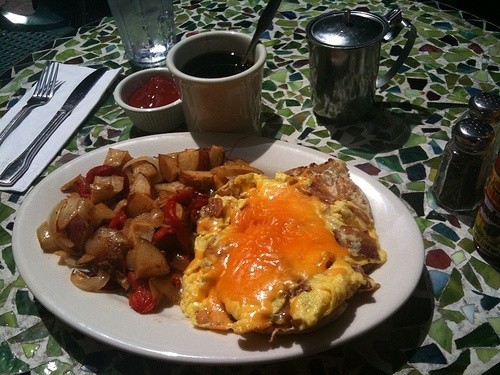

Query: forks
[0,61,60,146]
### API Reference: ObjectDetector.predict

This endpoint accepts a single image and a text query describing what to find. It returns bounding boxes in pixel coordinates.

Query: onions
[37,158,263,291]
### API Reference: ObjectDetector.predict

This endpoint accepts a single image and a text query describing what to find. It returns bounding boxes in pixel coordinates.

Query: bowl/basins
[113,67,185,132]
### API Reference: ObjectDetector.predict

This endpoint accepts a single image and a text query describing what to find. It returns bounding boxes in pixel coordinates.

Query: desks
[0,0,500,375]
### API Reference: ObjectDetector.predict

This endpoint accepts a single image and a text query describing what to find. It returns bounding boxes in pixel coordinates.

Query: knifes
[0,67,110,186]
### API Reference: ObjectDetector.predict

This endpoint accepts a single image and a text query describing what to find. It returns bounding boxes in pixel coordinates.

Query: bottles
[472,151,500,261]
[428,117,495,213]
[466,93,500,146]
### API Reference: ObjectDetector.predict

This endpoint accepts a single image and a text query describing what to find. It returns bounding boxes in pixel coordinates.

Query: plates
[11,131,426,366]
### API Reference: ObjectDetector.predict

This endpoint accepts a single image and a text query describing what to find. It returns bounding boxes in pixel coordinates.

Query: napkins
[0,62,123,193]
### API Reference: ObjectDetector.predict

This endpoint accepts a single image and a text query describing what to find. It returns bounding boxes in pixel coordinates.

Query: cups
[108,0,177,68]
[305,7,417,122]
[165,31,267,134]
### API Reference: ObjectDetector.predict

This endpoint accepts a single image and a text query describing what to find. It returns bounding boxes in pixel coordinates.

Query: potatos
[91,144,265,308]
